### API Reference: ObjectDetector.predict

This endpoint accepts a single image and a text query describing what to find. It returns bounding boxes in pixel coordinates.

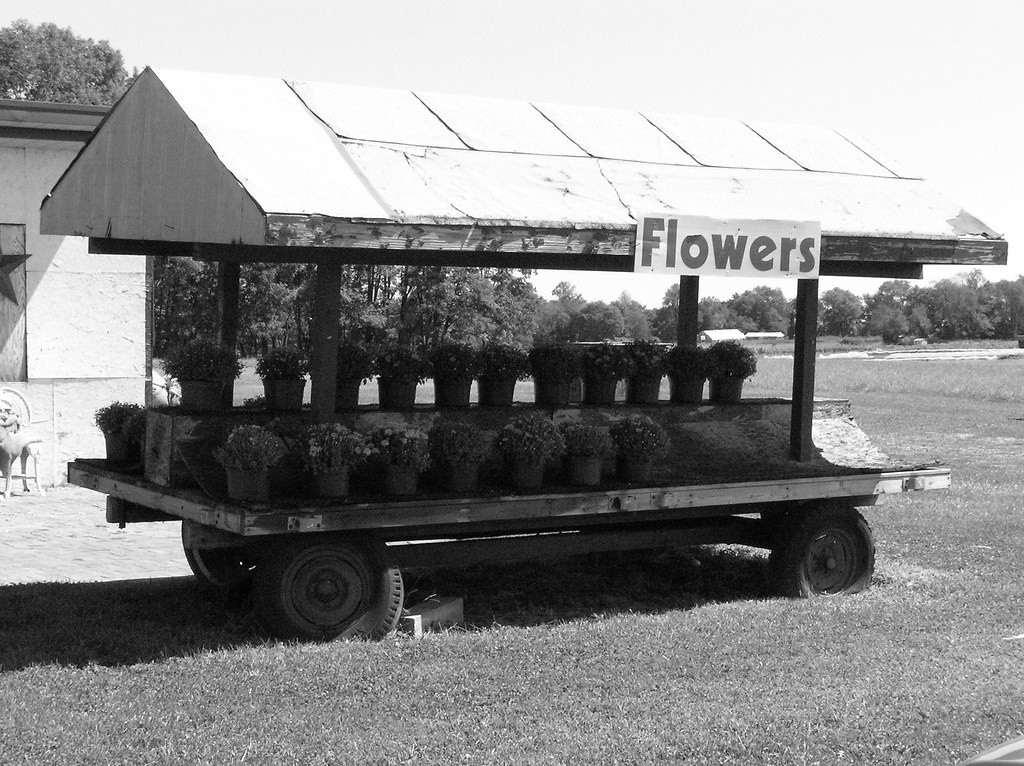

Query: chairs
[0,387,45,498]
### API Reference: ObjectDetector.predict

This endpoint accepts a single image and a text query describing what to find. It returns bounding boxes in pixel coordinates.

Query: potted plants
[96,341,760,503]
